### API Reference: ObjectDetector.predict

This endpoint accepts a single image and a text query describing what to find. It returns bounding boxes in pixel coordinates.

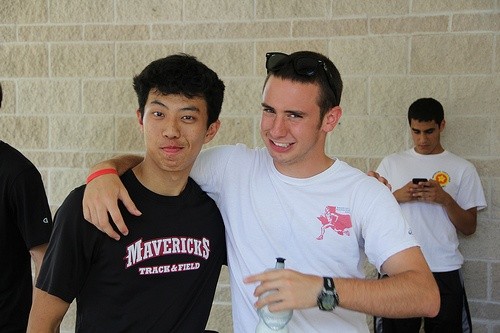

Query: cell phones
[412,178,427,187]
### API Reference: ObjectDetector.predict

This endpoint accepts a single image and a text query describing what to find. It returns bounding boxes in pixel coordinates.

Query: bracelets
[86,168,120,183]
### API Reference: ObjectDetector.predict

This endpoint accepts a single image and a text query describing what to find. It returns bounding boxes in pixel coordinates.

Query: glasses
[266,52,339,106]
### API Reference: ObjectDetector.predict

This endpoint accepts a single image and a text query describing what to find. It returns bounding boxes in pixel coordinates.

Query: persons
[82,53,440,333]
[0,85,62,333]
[26,53,228,333]
[374,98,487,332]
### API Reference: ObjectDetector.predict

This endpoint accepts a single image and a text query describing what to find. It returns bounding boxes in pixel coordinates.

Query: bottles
[256,258,294,333]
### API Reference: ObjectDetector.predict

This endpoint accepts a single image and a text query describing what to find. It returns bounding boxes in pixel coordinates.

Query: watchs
[317,276,339,313]
